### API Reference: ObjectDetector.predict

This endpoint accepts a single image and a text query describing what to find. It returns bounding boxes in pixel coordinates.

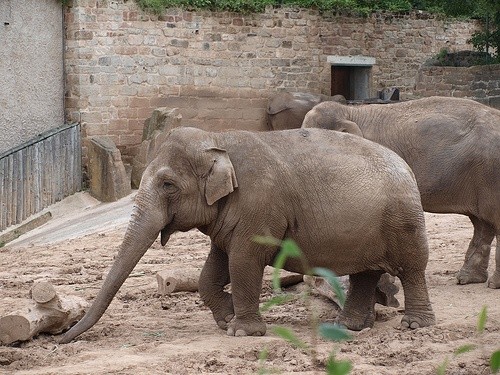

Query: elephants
[302,96,500,290]
[58,127,435,345]
[267,93,346,132]
